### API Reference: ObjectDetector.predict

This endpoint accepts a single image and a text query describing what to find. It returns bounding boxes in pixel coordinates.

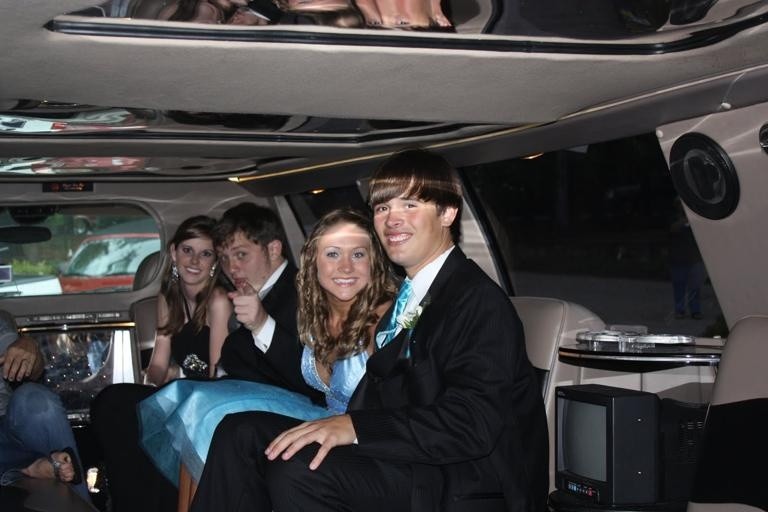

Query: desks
[556,339,725,393]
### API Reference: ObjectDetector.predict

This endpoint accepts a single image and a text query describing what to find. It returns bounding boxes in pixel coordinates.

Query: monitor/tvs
[554,383,708,512]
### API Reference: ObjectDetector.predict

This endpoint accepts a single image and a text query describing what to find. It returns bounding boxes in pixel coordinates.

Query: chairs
[677,313,764,508]
[129,292,159,385]
[510,292,607,496]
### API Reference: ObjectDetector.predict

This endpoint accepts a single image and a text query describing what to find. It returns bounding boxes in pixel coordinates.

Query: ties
[374,279,413,350]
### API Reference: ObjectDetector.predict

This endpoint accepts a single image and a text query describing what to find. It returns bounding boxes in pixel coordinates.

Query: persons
[0,306,95,511]
[89,214,235,510]
[129,0,460,38]
[184,144,529,512]
[136,205,400,512]
[211,197,326,410]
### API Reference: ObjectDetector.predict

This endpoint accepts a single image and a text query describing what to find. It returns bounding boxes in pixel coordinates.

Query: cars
[60,233,161,293]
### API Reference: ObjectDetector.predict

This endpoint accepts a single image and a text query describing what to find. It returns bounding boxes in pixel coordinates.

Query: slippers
[48,446,82,485]
